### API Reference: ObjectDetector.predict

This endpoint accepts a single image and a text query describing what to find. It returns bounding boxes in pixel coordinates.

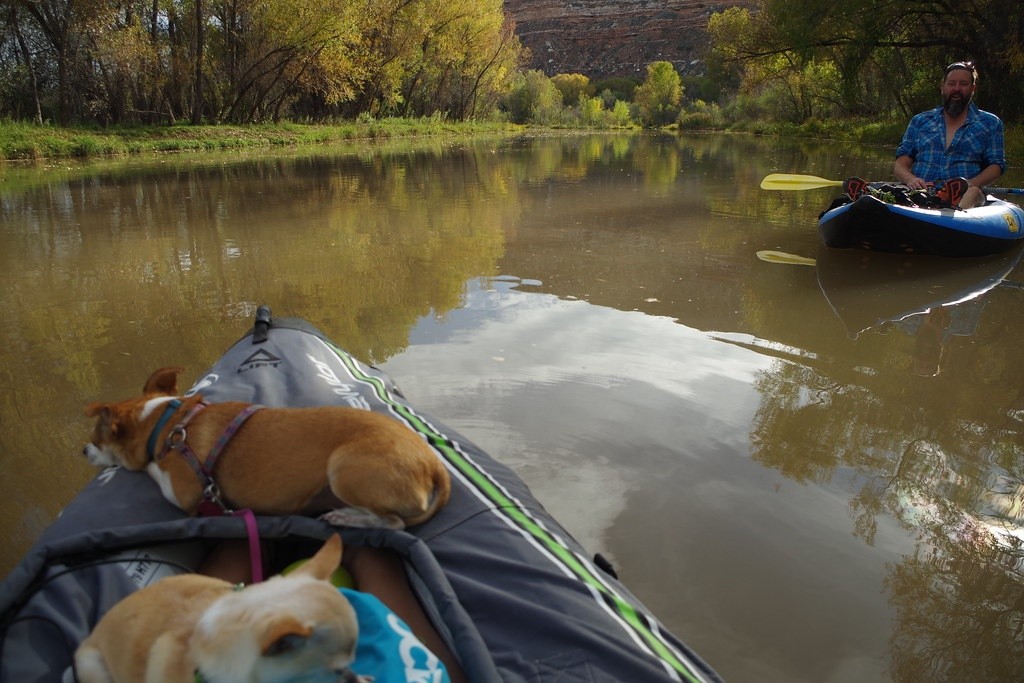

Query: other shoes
[939,177,967,205]
[844,177,869,200]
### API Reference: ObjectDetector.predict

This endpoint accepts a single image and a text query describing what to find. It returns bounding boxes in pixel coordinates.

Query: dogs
[75,532,359,676]
[82,368,453,527]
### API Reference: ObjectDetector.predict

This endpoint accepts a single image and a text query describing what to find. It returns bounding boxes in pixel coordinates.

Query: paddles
[760,174,1024,195]
[755,250,817,267]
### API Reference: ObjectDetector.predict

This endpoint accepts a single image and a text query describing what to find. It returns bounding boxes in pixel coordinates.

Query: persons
[845,61,1006,213]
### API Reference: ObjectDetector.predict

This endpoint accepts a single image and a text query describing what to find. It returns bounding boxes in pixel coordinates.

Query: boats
[805,245,1023,340]
[819,195,1024,253]
